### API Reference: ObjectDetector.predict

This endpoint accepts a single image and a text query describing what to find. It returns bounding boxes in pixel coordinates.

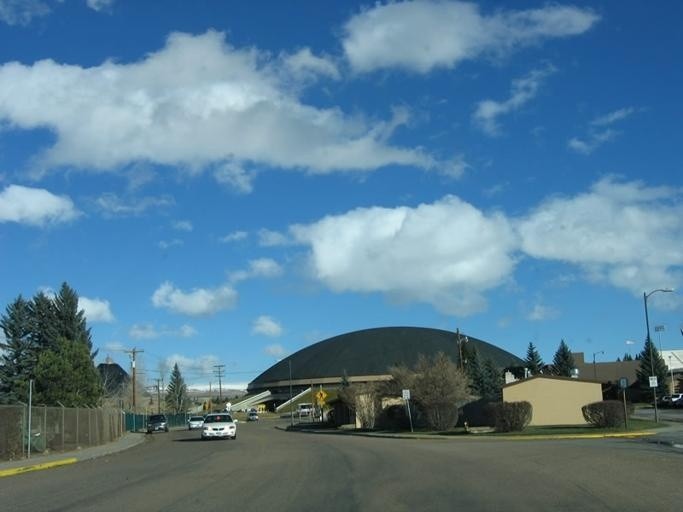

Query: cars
[246,411,258,422]
[188,416,206,432]
[652,393,683,409]
[199,413,240,440]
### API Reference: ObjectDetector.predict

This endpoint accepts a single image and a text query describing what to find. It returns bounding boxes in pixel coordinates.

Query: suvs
[145,413,170,435]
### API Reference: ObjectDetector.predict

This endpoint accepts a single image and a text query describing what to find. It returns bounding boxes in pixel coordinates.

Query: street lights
[592,351,605,378]
[454,325,471,372]
[642,288,675,422]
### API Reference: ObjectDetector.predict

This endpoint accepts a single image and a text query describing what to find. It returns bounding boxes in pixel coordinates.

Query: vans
[296,402,316,416]
